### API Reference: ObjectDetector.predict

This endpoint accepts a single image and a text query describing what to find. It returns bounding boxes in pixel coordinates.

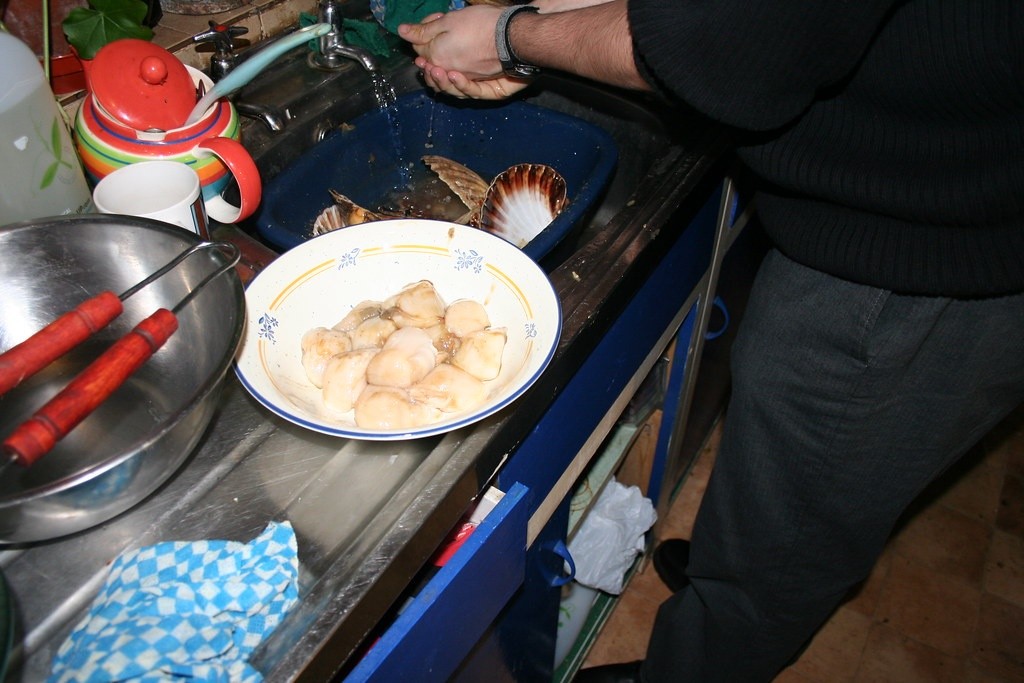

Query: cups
[93,161,208,238]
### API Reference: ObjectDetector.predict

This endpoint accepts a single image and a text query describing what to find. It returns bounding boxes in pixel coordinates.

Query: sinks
[225,42,667,278]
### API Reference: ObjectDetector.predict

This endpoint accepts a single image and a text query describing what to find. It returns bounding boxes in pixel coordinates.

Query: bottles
[0,29,94,225]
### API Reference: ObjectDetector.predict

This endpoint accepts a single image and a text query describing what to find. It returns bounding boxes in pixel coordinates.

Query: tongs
[0,241,241,469]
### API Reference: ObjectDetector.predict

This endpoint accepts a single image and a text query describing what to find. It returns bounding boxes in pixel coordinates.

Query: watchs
[495,4,544,79]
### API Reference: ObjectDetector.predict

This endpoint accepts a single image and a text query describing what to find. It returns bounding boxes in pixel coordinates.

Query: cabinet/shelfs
[335,155,777,683]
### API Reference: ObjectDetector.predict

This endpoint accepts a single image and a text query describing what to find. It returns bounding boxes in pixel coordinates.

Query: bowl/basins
[257,87,619,263]
[0,213,247,546]
[73,38,263,224]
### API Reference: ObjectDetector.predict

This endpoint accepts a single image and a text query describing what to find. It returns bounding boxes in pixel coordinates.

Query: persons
[397,0,1024,683]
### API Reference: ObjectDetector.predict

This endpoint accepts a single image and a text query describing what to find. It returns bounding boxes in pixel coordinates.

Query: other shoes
[573,658,642,683]
[654,539,695,592]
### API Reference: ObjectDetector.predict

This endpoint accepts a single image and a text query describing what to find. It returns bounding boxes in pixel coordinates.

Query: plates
[231,219,563,441]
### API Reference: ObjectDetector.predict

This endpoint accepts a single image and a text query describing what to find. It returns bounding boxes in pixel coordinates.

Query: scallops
[311,154,572,251]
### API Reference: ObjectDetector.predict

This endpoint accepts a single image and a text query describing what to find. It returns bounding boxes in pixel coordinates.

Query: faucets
[193,20,287,132]
[305,0,381,72]
